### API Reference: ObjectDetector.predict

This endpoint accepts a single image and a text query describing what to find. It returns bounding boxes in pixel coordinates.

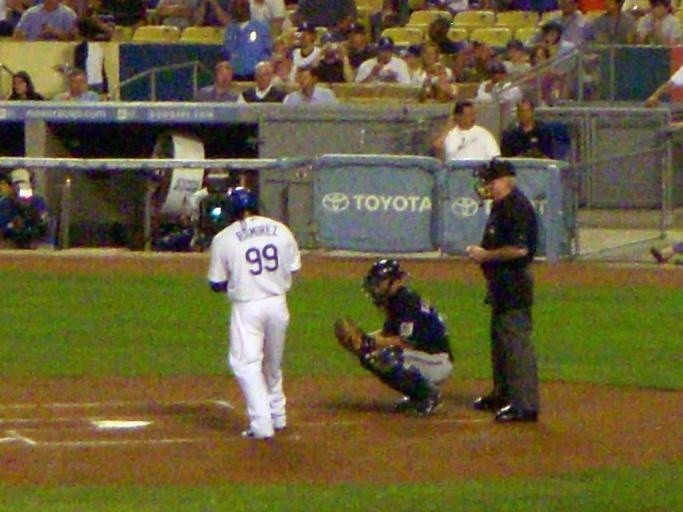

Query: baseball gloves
[334,316,360,353]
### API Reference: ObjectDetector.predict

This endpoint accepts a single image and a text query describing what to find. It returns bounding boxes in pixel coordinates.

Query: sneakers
[393,392,419,412]
[472,390,513,413]
[494,402,539,422]
[412,391,450,417]
[242,427,274,445]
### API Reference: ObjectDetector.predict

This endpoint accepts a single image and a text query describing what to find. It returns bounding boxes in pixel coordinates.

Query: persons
[206,186,302,440]
[334,259,453,418]
[464,160,541,423]
[0,0,683,262]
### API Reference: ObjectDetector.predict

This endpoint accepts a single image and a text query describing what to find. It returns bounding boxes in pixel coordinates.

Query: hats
[486,63,505,73]
[8,168,32,184]
[376,38,392,48]
[297,21,314,33]
[474,159,517,185]
[320,33,338,44]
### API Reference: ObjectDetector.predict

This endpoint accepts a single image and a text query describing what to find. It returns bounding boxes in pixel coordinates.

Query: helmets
[365,260,401,285]
[224,186,258,216]
